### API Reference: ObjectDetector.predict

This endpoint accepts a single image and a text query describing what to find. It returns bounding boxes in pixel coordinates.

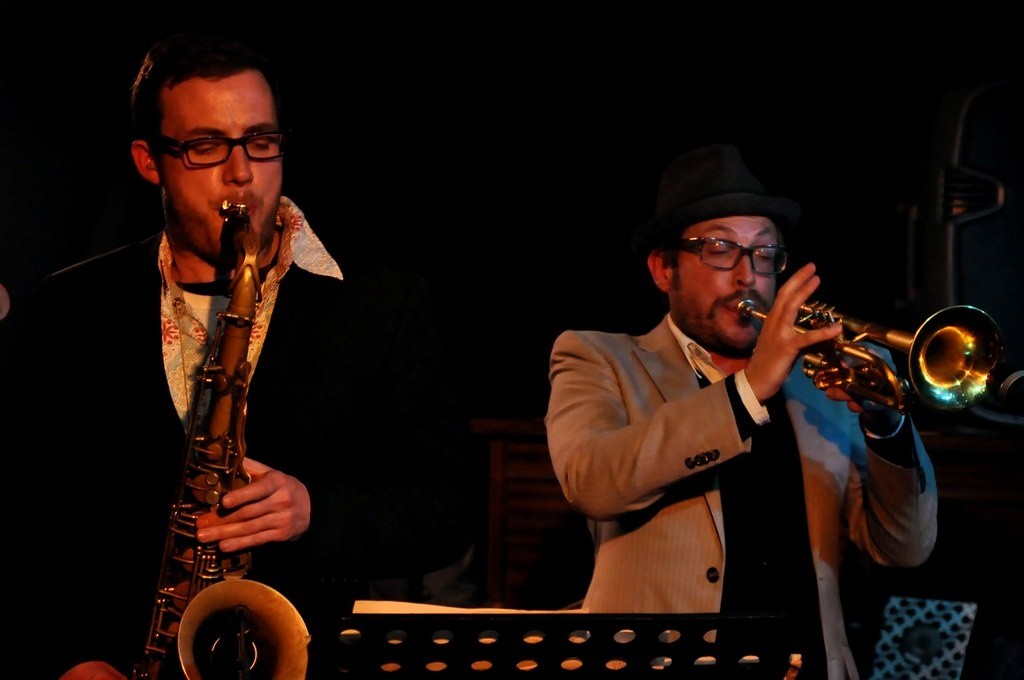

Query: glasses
[146,129,290,165]
[666,235,788,274]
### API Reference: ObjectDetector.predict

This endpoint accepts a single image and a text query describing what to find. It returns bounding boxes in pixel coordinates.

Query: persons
[544,145,938,680]
[0,35,399,680]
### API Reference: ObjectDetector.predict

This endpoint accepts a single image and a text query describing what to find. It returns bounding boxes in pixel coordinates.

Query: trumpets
[736,299,1011,422]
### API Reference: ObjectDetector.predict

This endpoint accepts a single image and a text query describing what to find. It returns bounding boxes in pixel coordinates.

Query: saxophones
[125,196,311,680]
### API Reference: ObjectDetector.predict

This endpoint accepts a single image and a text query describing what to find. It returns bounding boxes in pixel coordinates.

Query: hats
[629,144,798,255]
